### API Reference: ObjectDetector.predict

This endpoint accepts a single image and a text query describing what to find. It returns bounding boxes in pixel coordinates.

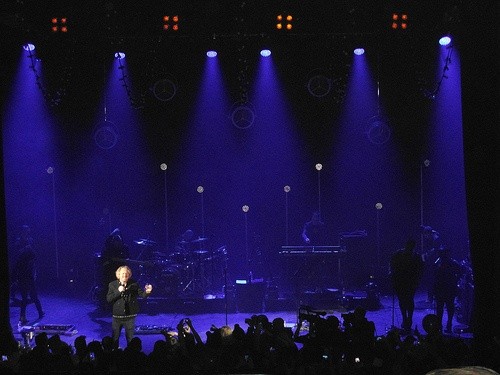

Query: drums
[161,264,194,294]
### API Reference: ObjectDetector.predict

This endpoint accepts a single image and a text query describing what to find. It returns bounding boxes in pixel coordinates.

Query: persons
[302,210,327,251]
[0,288,500,375]
[231,255,267,297]
[106,265,153,351]
[101,233,128,292]
[391,240,423,334]
[14,226,44,326]
[178,230,194,250]
[431,247,462,329]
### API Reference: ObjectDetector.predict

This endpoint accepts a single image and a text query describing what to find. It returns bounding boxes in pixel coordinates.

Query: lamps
[242,202,249,261]
[160,162,168,250]
[420,159,430,255]
[47,166,59,279]
[375,203,382,261]
[197,186,204,238]
[315,163,323,243]
[283,185,291,245]
[43,0,423,150]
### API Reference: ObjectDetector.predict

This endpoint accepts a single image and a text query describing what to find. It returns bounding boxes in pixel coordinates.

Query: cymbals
[191,237,207,242]
[193,251,208,253]
[139,238,155,243]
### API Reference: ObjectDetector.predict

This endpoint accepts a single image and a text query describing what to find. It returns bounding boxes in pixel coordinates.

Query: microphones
[121,282,125,295]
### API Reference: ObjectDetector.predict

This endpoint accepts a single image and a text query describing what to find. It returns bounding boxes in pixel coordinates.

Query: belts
[113,314,137,318]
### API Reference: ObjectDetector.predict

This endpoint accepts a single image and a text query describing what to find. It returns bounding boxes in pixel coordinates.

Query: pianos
[279,246,346,292]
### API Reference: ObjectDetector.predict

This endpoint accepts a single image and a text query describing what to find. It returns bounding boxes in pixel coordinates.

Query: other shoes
[444,328,452,334]
[40,310,45,316]
[402,320,413,328]
[17,321,25,328]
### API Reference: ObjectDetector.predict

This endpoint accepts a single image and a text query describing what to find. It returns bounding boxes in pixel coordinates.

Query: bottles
[249,271,253,281]
[161,331,179,347]
